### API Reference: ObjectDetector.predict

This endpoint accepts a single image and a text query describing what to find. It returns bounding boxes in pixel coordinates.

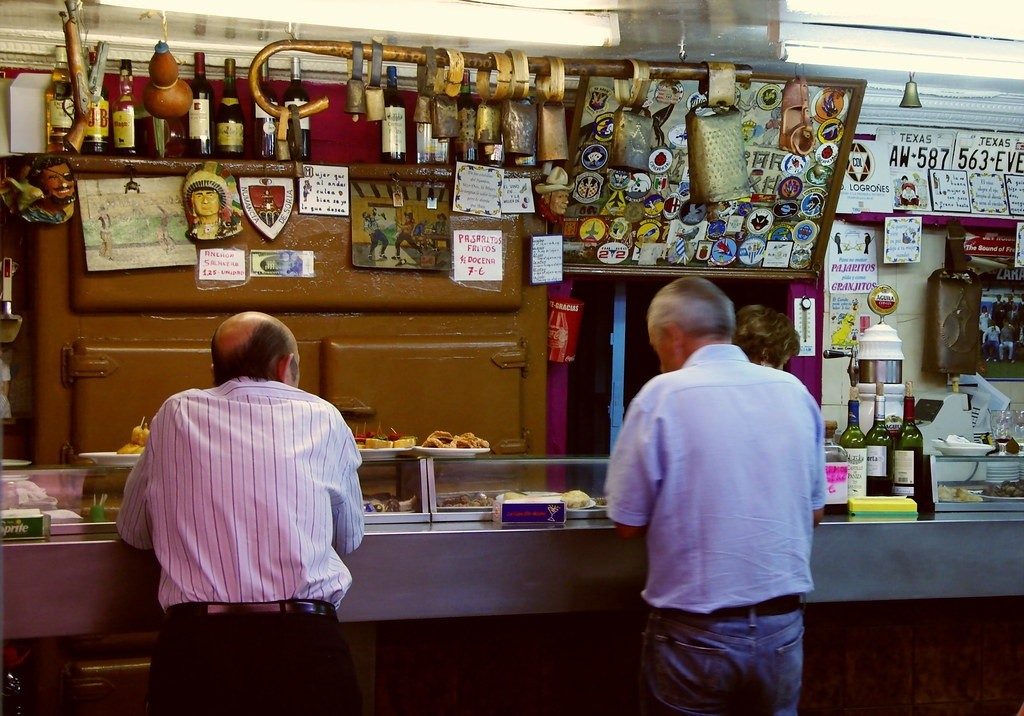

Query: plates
[356,447,415,461]
[931,439,1024,504]
[413,446,491,457]
[78,449,142,466]
[433,489,615,514]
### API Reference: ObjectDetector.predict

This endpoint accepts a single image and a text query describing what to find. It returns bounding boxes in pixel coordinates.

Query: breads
[560,490,590,509]
[503,492,526,498]
[358,434,417,449]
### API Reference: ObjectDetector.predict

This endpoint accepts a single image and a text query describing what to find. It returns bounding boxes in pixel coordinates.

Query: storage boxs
[492,500,567,525]
[850,497,918,517]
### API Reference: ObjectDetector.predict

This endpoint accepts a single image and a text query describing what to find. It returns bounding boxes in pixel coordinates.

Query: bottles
[380,67,548,167]
[251,56,282,160]
[215,58,245,161]
[821,379,923,516]
[182,50,217,159]
[45,44,81,153]
[110,59,144,157]
[283,55,310,161]
[82,50,110,155]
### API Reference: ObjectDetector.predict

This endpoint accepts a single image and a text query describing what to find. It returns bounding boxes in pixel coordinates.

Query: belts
[716,595,801,618]
[172,601,338,616]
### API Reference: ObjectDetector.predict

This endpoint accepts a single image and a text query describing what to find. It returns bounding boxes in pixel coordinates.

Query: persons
[604,274,824,716]
[116,312,367,716]
[733,301,802,371]
[978,292,1024,364]
[183,170,243,240]
[19,153,77,225]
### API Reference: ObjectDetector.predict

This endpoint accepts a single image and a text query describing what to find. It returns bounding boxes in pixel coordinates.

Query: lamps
[85,0,621,48]
[766,21,1024,80]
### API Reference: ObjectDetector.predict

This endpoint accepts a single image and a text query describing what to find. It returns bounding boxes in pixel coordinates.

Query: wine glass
[989,409,1024,455]
